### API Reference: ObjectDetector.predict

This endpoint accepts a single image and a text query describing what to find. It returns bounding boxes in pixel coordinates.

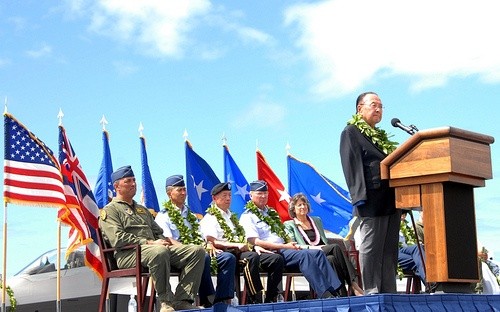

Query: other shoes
[321,287,342,299]
[425,282,438,294]
[266,287,279,304]
[214,293,226,305]
[250,291,263,304]
[171,300,200,311]
[160,300,176,312]
[350,281,365,296]
[199,295,217,308]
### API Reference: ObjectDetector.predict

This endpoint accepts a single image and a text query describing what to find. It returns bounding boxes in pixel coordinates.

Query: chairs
[280,272,316,303]
[239,272,267,305]
[396,273,421,294]
[96,228,181,312]
[325,237,363,296]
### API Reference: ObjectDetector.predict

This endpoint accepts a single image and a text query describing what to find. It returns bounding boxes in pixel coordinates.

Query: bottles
[127,295,137,312]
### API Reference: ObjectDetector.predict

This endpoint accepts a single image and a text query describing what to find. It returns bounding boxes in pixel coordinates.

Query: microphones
[391,117,415,135]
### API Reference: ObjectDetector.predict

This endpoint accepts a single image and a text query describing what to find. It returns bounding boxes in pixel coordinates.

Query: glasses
[359,102,382,110]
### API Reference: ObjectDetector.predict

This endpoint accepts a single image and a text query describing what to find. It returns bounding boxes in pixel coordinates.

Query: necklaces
[396,242,405,281]
[347,114,400,155]
[293,214,320,246]
[205,203,262,272]
[244,200,292,244]
[163,200,218,275]
[399,219,418,246]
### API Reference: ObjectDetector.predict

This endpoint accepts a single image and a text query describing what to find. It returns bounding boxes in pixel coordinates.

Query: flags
[58,125,104,281]
[3,113,75,238]
[185,141,293,223]
[140,137,160,219]
[287,155,354,238]
[93,131,116,209]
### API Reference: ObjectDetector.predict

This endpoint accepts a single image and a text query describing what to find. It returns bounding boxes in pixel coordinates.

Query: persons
[349,206,500,294]
[283,193,363,297]
[238,181,342,300]
[98,166,204,312]
[340,92,411,296]
[199,182,284,304]
[154,175,236,308]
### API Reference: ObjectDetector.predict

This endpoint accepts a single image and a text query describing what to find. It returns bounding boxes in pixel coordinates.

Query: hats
[212,181,232,196]
[111,165,134,184]
[250,180,268,191]
[166,175,185,187]
